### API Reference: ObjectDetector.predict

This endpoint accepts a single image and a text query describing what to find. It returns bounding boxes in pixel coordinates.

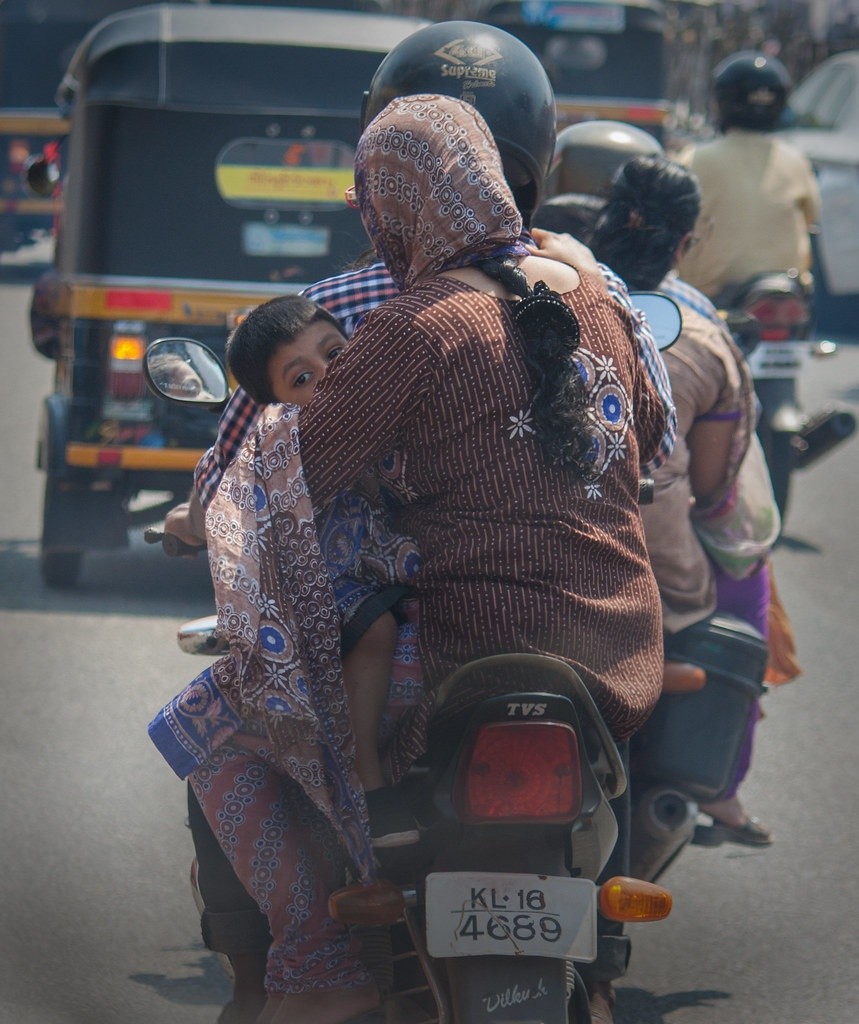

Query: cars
[771,50,859,301]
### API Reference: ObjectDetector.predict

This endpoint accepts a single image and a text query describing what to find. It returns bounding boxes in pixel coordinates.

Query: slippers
[693,818,775,847]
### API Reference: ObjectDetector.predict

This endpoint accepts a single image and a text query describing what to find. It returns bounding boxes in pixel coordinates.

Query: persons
[144,91,677,1022]
[672,50,827,301]
[165,16,671,1024]
[147,352,222,404]
[226,296,426,852]
[576,158,774,852]
[550,116,729,334]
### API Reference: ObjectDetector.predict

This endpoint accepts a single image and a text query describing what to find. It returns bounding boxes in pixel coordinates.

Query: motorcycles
[145,293,762,1024]
[487,0,691,157]
[0,1,109,256]
[20,3,508,589]
[717,267,857,539]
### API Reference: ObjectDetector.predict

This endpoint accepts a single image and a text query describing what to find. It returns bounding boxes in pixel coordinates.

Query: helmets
[709,51,793,134]
[531,119,669,241]
[361,19,561,232]
